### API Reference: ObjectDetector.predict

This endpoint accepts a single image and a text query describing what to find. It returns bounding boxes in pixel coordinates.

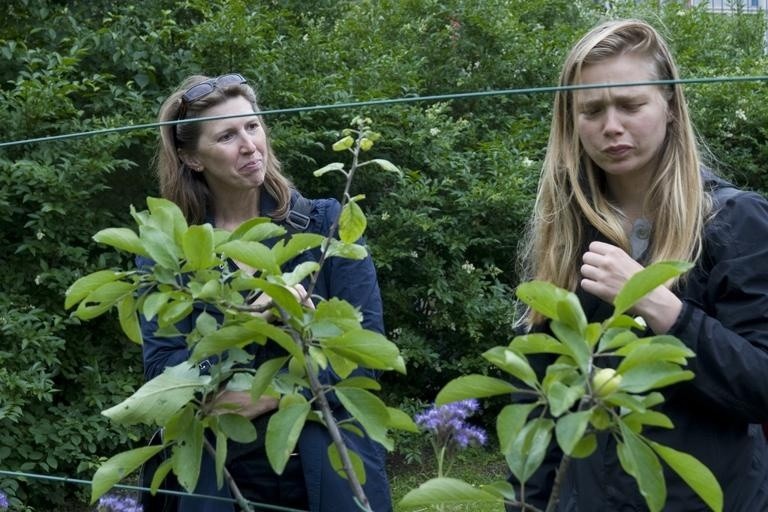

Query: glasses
[178,73,247,120]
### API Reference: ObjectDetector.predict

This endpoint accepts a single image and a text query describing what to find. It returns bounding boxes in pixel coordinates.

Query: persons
[503,17,767,512]
[136,73,393,512]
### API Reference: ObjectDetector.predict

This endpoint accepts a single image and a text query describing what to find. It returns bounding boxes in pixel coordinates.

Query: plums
[591,368,624,397]
[287,352,318,383]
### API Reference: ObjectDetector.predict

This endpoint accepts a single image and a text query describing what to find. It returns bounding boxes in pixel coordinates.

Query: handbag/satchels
[136,428,180,512]
